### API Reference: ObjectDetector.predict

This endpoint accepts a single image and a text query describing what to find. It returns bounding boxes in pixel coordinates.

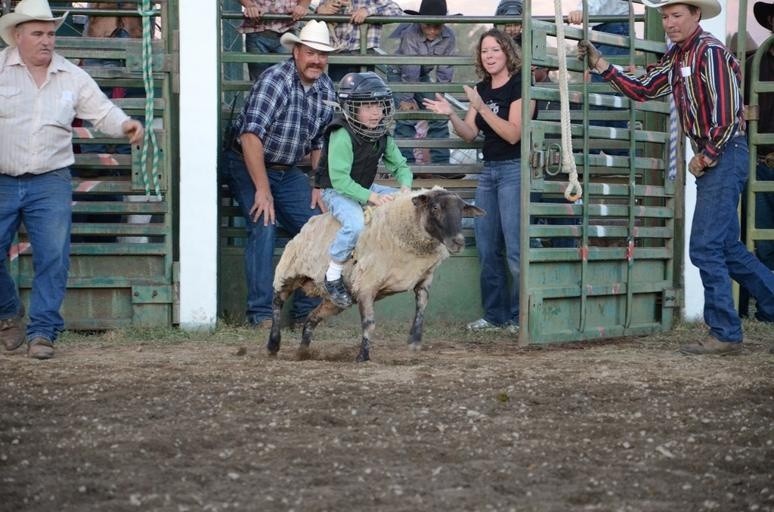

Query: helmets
[336,70,395,143]
[493,0,522,37]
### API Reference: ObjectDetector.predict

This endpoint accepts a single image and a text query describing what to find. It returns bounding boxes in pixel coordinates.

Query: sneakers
[466,318,519,336]
[324,274,353,309]
[680,335,744,356]
[28,336,54,360]
[0,302,25,350]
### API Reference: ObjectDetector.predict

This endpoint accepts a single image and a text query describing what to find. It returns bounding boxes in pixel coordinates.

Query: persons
[0,0,774,358]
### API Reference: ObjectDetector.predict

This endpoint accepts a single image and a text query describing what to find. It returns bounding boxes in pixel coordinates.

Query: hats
[640,0,721,20]
[404,0,462,16]
[0,0,69,47]
[754,0,774,31]
[280,19,345,52]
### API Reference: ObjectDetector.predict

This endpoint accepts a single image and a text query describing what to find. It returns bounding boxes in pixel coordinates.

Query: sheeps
[266,183,486,363]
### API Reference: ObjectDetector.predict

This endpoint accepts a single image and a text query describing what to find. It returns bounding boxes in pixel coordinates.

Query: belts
[268,164,289,171]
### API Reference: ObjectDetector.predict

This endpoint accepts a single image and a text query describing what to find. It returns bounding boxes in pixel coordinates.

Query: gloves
[577,38,602,69]
[688,148,717,178]
